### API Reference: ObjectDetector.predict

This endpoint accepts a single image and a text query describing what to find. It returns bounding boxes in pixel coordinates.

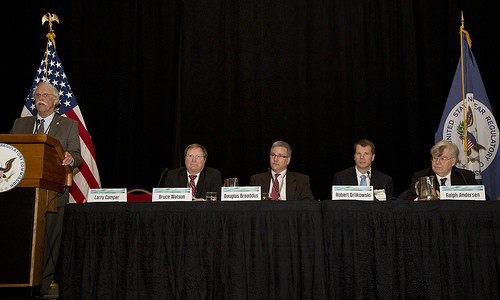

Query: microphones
[454,169,467,185]
[157,168,168,188]
[268,167,281,200]
[36,119,40,134]
[367,171,373,186]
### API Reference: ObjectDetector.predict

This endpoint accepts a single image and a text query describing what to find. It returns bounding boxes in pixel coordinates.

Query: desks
[54,200,500,300]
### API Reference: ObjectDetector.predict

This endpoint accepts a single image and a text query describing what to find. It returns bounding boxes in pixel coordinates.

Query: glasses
[34,92,57,98]
[185,153,205,160]
[430,155,450,163]
[356,151,372,158]
[270,152,288,159]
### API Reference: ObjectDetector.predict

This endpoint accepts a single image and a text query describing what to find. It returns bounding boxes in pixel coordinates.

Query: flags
[435,27,500,200]
[21,32,101,203]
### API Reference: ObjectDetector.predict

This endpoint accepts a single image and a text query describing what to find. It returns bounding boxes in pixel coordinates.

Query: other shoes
[42,281,59,299]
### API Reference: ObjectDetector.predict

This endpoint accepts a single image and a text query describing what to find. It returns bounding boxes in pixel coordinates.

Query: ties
[271,174,281,200]
[440,178,447,186]
[38,119,45,134]
[189,175,197,198]
[360,175,367,186]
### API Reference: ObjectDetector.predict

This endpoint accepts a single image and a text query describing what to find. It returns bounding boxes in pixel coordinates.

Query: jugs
[224,177,238,187]
[414,177,439,201]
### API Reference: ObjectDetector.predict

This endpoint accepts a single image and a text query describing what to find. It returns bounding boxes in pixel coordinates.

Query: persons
[251,141,314,202]
[405,140,489,201]
[166,144,222,200]
[333,139,393,198]
[9,83,82,297]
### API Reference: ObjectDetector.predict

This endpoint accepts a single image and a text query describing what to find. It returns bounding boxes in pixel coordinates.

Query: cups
[263,193,272,200]
[206,192,217,201]
